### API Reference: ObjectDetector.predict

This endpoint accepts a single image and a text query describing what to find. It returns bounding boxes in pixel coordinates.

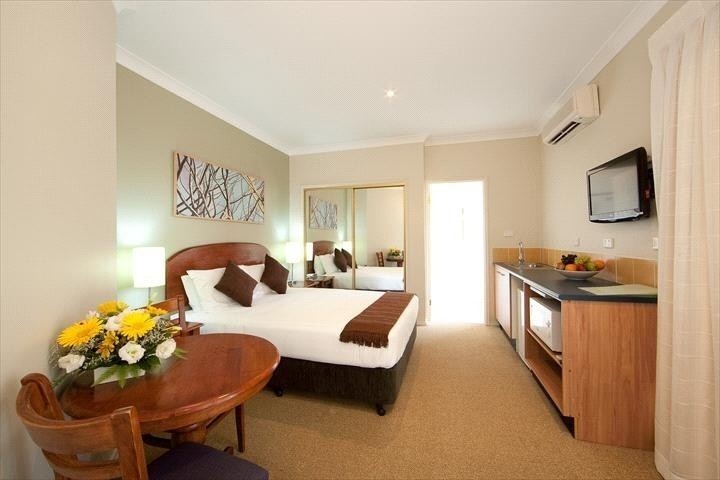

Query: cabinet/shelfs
[522,280,657,452]
[494,263,520,340]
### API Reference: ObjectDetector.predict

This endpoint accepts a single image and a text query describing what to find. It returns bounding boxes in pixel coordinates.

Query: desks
[60,332,280,454]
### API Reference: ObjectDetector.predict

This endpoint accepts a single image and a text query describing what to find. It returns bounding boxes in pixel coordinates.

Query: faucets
[518,242,526,264]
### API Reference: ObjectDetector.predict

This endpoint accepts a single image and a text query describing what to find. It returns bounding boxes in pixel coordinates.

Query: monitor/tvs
[586,146,655,223]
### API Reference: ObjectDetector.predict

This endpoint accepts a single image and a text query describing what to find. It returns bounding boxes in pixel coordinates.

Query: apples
[565,260,604,271]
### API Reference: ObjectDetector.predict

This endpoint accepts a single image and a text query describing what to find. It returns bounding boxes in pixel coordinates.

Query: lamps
[285,241,303,286]
[133,247,167,306]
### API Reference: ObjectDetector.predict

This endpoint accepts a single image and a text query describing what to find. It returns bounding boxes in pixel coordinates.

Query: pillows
[180,252,290,311]
[314,247,358,275]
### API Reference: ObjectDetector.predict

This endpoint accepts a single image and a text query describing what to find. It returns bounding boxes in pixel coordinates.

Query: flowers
[47,299,187,400]
[386,245,403,257]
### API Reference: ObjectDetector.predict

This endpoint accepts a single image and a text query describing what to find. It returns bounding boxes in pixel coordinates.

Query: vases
[81,365,146,385]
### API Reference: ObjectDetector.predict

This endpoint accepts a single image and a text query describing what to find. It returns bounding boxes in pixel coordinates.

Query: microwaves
[528,295,561,355]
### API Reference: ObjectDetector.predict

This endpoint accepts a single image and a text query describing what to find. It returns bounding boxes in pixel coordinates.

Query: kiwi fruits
[555,262,565,270]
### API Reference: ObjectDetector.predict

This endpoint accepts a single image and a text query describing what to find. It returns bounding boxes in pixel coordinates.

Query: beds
[165,242,420,417]
[312,240,405,291]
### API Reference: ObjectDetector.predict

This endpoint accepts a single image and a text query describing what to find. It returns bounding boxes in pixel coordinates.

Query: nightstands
[304,274,335,288]
[172,321,204,337]
[288,281,319,288]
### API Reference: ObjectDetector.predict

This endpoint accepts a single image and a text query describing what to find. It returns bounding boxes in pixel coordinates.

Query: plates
[552,267,601,282]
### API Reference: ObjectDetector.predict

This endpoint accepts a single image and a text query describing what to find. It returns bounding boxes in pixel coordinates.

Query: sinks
[507,262,548,271]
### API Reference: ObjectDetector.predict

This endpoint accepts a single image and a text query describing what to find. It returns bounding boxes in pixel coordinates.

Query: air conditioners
[541,82,599,146]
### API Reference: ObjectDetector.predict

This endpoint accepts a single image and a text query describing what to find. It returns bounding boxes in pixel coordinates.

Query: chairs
[133,294,246,455]
[16,373,270,480]
[375,251,385,267]
